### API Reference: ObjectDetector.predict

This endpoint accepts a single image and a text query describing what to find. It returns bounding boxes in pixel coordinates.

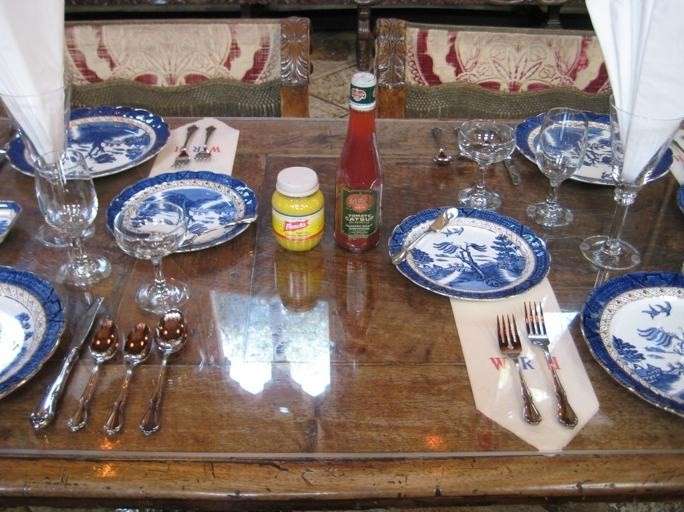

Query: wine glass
[580,96,683,272]
[457,119,516,211]
[37,149,113,290]
[527,108,587,230]
[0,85,96,247]
[114,201,192,316]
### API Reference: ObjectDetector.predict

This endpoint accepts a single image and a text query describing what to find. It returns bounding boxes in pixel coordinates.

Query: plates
[517,109,674,186]
[5,107,168,180]
[389,204,551,301]
[105,171,258,254]
[583,271,684,422]
[0,264,62,397]
[0,197,20,243]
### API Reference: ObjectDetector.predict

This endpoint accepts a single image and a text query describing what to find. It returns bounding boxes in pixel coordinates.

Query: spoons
[429,126,451,164]
[175,215,257,254]
[69,308,191,433]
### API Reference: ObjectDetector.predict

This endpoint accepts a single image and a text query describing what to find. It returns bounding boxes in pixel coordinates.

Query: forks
[494,314,541,427]
[194,127,215,159]
[523,302,578,428]
[171,124,198,171]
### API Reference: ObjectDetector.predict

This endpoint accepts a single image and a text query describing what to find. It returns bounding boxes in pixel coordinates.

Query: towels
[584,0,684,182]
[0,0,66,166]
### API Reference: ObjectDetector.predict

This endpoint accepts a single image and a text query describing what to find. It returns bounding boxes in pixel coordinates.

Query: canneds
[273,247,325,313]
[272,165,324,253]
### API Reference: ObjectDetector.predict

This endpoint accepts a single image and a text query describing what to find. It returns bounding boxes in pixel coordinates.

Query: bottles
[333,252,379,360]
[333,73,383,251]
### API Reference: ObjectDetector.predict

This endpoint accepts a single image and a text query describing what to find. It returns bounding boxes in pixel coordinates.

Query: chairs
[62,16,310,117]
[374,18,613,121]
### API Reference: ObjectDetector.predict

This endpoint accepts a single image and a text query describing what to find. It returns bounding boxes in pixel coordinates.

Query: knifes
[503,157,521,185]
[26,297,104,430]
[392,208,458,264]
